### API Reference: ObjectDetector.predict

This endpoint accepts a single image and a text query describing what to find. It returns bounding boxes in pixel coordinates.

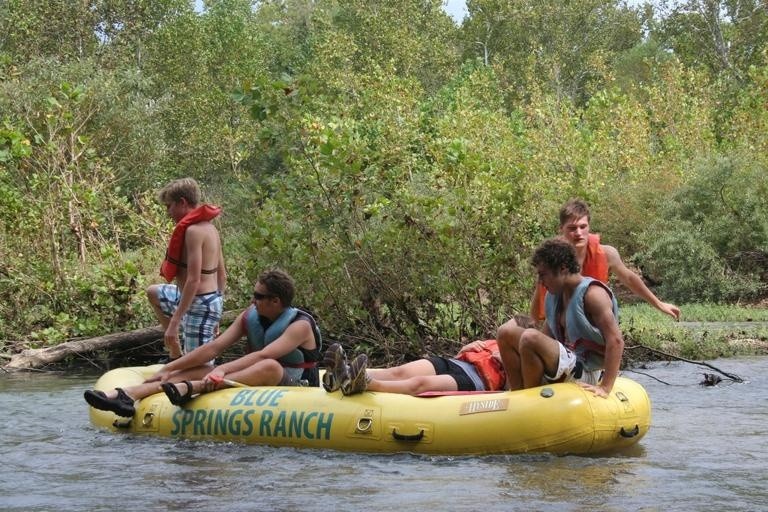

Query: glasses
[253,292,273,300]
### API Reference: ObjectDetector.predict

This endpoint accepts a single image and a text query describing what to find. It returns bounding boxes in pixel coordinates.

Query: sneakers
[342,354,368,396]
[323,343,348,393]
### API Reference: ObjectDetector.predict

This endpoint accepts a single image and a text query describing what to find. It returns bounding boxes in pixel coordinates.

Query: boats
[88,363,652,457]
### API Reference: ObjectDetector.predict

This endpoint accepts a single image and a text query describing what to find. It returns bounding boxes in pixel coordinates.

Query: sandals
[161,380,193,404]
[84,388,135,418]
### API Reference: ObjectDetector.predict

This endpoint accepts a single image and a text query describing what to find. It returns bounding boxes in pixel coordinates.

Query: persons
[146,178,228,368]
[322,313,536,397]
[495,235,626,399]
[83,268,322,418]
[530,199,683,324]
